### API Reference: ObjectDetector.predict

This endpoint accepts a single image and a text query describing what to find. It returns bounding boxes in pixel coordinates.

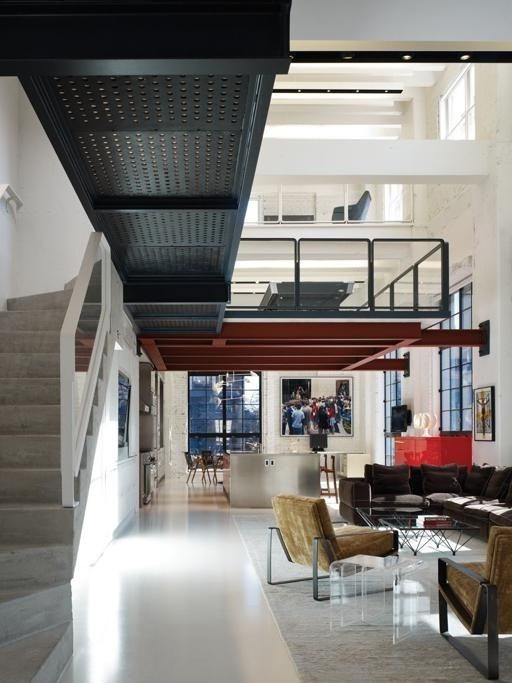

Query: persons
[282,385,351,434]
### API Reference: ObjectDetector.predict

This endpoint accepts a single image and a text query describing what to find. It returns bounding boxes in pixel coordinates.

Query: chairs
[437,525,512,683]
[331,189,372,222]
[184,449,224,485]
[266,491,399,600]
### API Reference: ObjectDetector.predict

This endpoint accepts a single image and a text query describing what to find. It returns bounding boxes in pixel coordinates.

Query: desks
[394,433,471,471]
[316,451,367,504]
[258,280,355,311]
[357,506,480,556]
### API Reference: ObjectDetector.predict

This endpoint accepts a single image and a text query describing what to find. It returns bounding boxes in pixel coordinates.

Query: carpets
[231,509,511,680]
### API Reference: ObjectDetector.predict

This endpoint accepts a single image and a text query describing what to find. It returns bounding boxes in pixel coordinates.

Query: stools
[329,554,432,644]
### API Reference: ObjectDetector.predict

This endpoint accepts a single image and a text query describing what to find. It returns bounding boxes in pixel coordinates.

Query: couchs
[338,463,512,538]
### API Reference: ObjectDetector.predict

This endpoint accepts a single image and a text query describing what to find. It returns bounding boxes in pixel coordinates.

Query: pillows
[370,462,410,494]
[465,463,510,501]
[420,463,461,494]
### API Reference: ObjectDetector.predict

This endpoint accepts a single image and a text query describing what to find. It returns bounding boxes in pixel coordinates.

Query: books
[416,515,452,528]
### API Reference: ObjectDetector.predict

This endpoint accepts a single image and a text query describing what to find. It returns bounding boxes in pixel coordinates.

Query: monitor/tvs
[391,405,411,433]
[309,434,329,450]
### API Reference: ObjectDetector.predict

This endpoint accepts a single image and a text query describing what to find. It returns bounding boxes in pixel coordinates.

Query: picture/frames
[472,385,495,441]
[280,375,355,436]
